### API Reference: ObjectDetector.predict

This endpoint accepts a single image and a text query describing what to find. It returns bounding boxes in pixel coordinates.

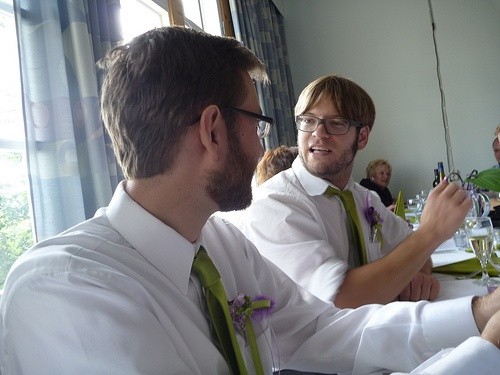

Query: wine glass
[465,217,500,287]
[407,194,425,224]
[487,228,500,288]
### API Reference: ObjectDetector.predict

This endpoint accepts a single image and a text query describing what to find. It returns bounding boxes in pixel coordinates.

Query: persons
[256,145,299,186]
[239,74,472,309]
[482,124,500,225]
[0,28,500,375]
[359,159,396,210]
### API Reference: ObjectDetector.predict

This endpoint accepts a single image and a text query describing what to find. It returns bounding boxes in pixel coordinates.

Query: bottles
[433,162,445,188]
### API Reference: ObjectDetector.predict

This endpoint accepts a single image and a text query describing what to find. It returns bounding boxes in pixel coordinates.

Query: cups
[452,189,491,252]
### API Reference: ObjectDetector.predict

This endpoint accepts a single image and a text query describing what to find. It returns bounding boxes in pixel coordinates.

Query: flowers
[230,292,276,335]
[365,207,386,244]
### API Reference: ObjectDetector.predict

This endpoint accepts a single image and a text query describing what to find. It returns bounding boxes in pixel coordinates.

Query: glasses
[296,115,365,135]
[188,105,275,139]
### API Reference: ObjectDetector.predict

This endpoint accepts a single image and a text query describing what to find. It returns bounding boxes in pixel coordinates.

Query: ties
[323,185,367,266]
[191,244,249,375]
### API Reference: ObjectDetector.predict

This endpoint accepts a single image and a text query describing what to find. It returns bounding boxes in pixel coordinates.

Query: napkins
[430,251,500,279]
[394,191,406,222]
